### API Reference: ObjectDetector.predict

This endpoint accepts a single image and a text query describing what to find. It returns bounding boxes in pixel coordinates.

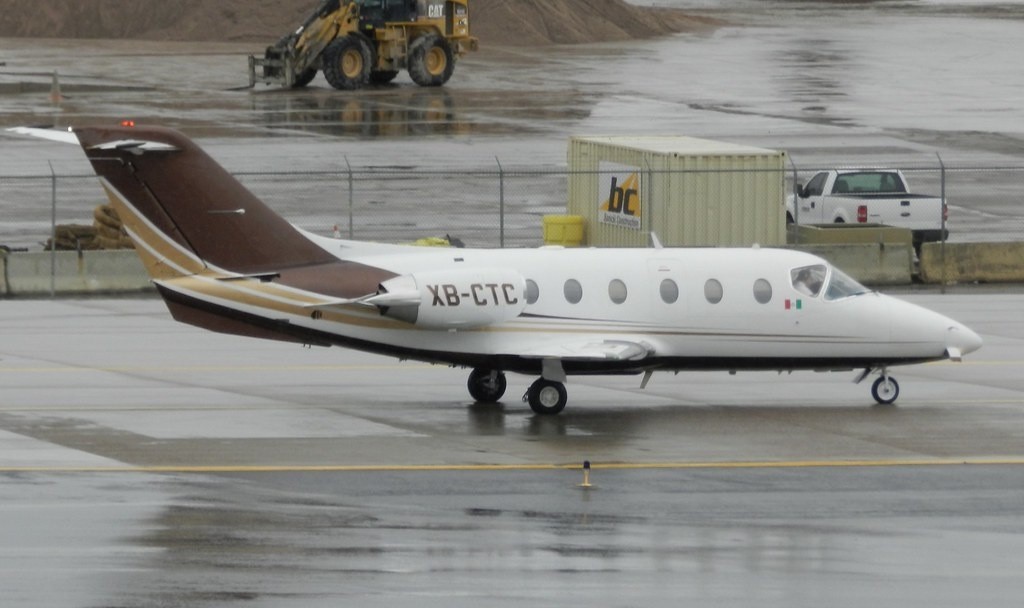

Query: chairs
[831,179,897,193]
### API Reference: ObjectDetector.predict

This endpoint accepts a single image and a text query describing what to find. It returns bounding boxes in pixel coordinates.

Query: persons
[793,269,817,296]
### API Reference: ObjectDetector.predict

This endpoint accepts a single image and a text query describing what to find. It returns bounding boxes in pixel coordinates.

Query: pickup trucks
[786,167,948,248]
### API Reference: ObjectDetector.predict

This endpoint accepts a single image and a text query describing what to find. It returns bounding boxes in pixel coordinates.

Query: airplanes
[7,125,985,416]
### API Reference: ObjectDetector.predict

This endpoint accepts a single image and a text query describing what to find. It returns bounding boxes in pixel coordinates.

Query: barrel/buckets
[542,214,584,249]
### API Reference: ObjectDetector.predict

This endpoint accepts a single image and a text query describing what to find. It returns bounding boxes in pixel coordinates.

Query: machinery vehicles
[225,0,480,92]
